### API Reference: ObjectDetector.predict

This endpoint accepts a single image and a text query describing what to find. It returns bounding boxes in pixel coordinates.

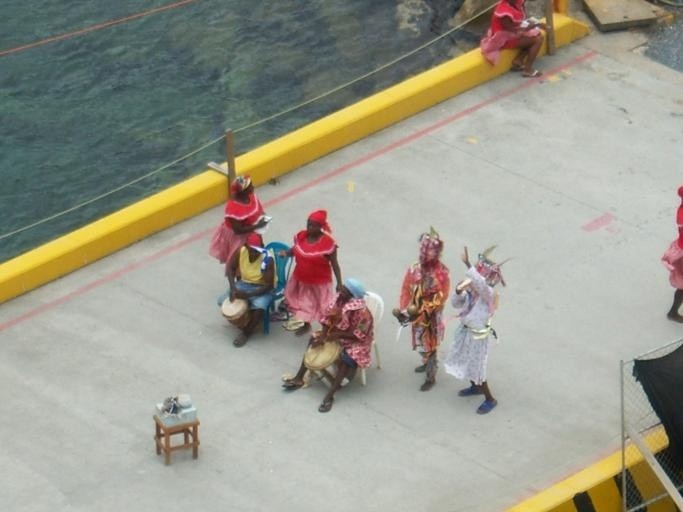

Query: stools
[154,414,200,464]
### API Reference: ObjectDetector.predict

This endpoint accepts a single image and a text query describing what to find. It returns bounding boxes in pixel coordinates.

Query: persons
[282,208,343,336]
[393,227,451,391]
[443,247,514,414]
[478,0,546,79]
[211,174,273,264]
[217,231,277,347]
[282,279,374,414]
[661,184,683,323]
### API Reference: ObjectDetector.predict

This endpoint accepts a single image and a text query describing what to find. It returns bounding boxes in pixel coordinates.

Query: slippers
[458,386,483,396]
[477,400,498,415]
[510,61,525,72]
[521,68,541,78]
[318,396,334,413]
[233,333,250,347]
[282,379,305,389]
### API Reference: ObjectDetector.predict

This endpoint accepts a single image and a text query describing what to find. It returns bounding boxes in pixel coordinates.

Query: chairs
[360,291,384,384]
[262,243,291,337]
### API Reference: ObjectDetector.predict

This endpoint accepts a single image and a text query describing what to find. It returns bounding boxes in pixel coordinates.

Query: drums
[303,337,357,390]
[220,296,252,330]
